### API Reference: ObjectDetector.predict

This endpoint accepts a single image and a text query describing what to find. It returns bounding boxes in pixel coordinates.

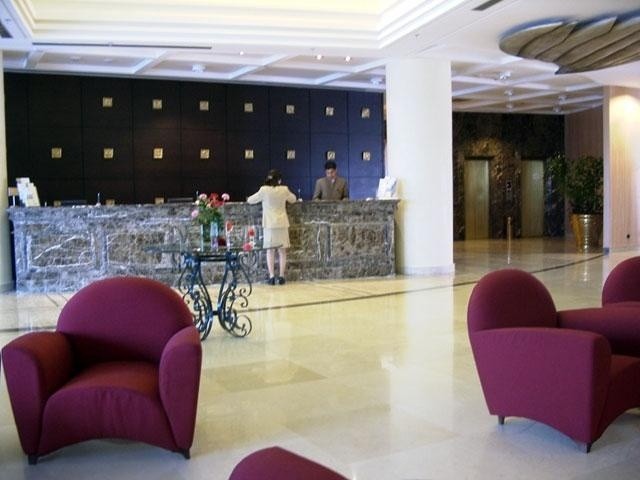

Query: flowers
[190,192,233,230]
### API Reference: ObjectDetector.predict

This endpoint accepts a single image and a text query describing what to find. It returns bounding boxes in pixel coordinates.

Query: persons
[312,161,349,202]
[247,171,296,285]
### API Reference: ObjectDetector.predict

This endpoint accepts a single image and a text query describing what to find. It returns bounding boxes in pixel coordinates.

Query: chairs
[602,256,640,307]
[467,268,640,453]
[1,277,202,465]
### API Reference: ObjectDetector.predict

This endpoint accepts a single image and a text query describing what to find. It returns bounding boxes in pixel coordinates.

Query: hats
[268,168,285,178]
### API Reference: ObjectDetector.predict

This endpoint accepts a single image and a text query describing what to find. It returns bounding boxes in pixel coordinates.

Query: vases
[210,221,219,252]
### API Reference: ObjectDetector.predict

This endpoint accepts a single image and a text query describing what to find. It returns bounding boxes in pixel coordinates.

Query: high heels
[279,277,285,284]
[268,278,275,285]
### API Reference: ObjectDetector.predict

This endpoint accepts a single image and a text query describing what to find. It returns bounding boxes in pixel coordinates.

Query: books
[15,176,40,207]
[376,176,398,200]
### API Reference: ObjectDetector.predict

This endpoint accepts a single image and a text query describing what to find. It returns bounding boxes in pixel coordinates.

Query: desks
[140,241,283,341]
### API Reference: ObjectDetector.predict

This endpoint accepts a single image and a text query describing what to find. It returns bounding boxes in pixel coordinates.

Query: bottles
[297,188,303,201]
[95,192,102,206]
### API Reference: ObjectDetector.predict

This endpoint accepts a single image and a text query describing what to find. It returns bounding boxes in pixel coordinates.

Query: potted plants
[545,149,603,255]
